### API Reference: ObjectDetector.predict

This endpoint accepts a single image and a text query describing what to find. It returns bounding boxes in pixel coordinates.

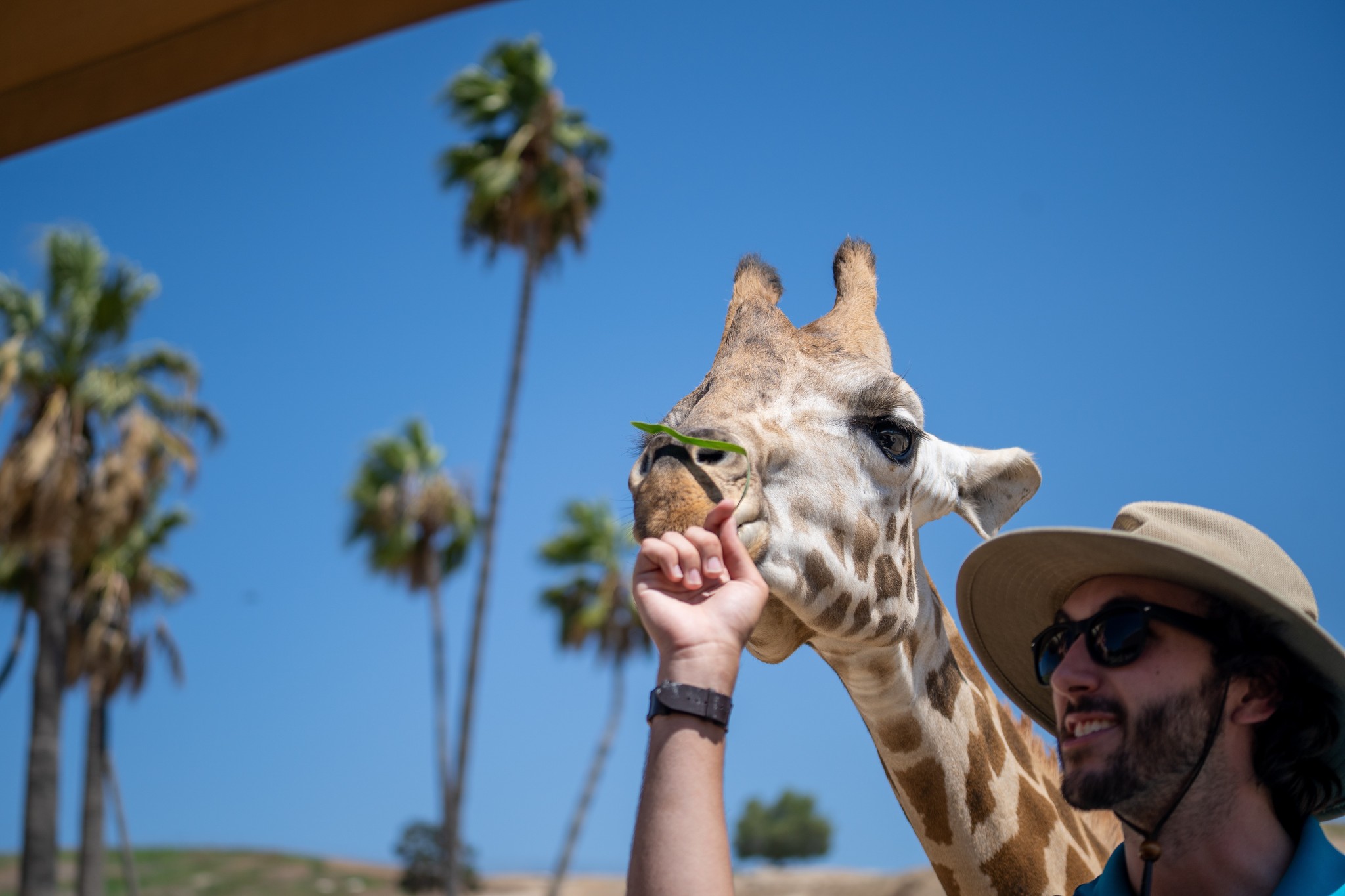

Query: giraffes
[627,235,1125,896]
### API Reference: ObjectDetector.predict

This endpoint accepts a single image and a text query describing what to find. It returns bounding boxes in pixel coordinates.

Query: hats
[954,499,1345,822]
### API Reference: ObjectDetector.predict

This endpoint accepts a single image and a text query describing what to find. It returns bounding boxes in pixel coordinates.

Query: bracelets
[642,683,733,731]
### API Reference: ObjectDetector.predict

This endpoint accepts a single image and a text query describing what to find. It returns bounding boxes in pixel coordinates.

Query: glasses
[1030,593,1222,689]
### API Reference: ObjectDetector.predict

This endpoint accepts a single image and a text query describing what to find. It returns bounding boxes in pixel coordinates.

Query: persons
[625,501,1345,896]
